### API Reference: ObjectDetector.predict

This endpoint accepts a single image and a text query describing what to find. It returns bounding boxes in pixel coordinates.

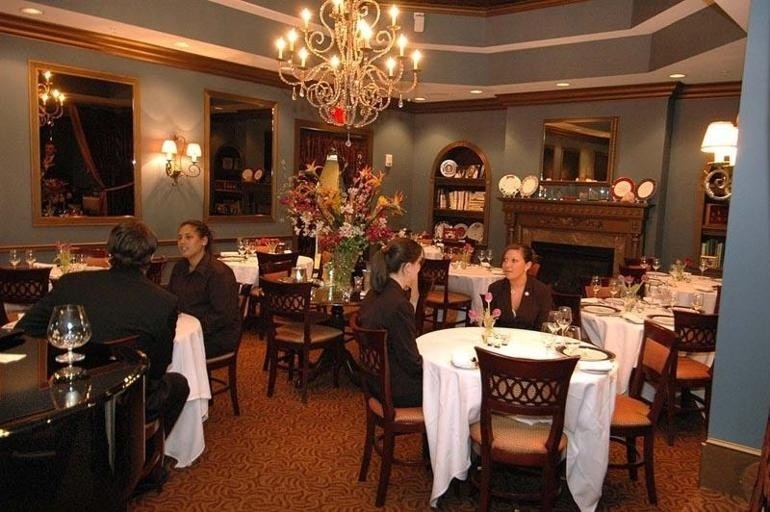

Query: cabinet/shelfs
[496,195,656,313]
[429,141,491,248]
[241,183,272,215]
[695,163,734,275]
[210,142,245,214]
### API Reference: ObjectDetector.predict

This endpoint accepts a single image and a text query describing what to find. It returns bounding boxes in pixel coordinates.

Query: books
[436,190,485,212]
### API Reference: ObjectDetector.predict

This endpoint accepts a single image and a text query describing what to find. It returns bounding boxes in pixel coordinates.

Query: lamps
[161,134,203,186]
[700,121,734,164]
[39,68,66,128]
[274,1,422,147]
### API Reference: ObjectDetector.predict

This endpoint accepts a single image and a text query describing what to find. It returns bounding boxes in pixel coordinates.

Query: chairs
[611,321,677,506]
[631,311,719,447]
[258,276,343,404]
[0,267,51,325]
[349,312,425,508]
[206,284,250,415]
[470,346,578,512]
[417,259,471,334]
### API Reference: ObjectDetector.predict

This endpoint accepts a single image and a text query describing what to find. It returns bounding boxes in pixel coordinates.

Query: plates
[519,175,539,196]
[440,160,458,177]
[453,223,468,240]
[467,222,484,240]
[434,220,452,240]
[240,167,254,182]
[662,304,706,316]
[253,168,264,181]
[578,302,621,318]
[647,313,676,328]
[498,174,522,196]
[635,177,658,200]
[610,177,636,201]
[557,345,618,364]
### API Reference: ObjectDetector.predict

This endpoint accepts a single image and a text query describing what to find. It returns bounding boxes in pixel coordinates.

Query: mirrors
[28,59,142,226]
[204,90,279,224]
[539,115,621,188]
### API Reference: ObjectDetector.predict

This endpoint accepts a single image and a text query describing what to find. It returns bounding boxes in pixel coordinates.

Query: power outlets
[386,154,393,167]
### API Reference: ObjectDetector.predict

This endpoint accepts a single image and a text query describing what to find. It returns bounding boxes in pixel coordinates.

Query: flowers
[671,258,692,271]
[274,160,408,250]
[616,274,650,292]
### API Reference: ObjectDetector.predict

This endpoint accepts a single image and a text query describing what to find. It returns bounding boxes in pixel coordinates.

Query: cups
[562,326,584,358]
[651,257,662,280]
[588,275,603,302]
[546,309,563,346]
[234,235,256,261]
[44,303,94,364]
[553,306,574,353]
[485,248,496,274]
[23,247,39,269]
[475,249,486,270]
[607,278,622,304]
[7,247,22,270]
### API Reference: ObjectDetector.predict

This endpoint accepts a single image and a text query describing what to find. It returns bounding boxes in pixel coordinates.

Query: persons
[42,141,70,178]
[12,218,189,496]
[483,244,554,330]
[170,220,242,359]
[355,237,433,471]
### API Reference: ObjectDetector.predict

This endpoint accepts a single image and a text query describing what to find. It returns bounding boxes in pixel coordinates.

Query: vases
[624,295,636,310]
[482,318,496,340]
[469,294,502,322]
[672,271,686,284]
[470,293,500,345]
[322,247,358,305]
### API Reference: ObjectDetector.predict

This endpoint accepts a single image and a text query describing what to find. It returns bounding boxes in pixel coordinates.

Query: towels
[297,256,313,279]
